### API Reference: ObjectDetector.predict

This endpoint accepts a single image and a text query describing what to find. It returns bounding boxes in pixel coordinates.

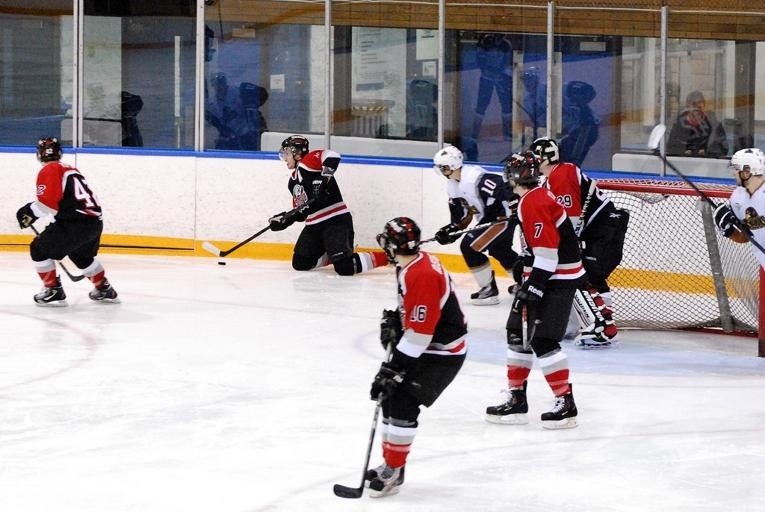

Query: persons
[121,92,144,146]
[527,137,630,349]
[653,90,729,159]
[433,146,525,305]
[715,148,765,275]
[267,135,388,276]
[363,215,468,498]
[205,80,269,150]
[375,33,601,171]
[212,76,243,112]
[485,149,587,430]
[15,136,119,307]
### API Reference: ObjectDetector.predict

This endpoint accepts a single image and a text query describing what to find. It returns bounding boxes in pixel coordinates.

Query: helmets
[433,145,464,175]
[504,152,540,182]
[726,148,765,176]
[210,68,227,85]
[521,66,539,80]
[37,136,61,161]
[375,216,420,253]
[278,135,309,162]
[527,137,560,162]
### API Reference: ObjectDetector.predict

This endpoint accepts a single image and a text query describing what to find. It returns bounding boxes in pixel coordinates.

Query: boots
[363,460,405,490]
[470,107,482,140]
[502,111,518,138]
[487,389,527,415]
[470,281,499,299]
[541,392,578,421]
[575,324,618,346]
[88,277,118,300]
[34,282,66,303]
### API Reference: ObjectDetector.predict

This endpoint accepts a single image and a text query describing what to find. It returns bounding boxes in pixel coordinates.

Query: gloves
[369,361,403,400]
[311,177,329,200]
[712,200,735,237]
[380,308,404,350]
[17,201,38,229]
[268,211,291,231]
[511,281,547,316]
[435,223,460,245]
